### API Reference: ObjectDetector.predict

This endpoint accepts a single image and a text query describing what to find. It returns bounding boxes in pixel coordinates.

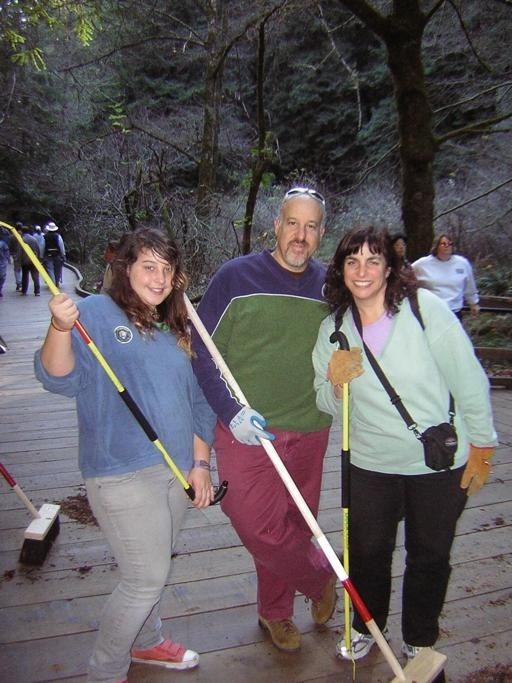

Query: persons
[189,186,347,654]
[0,218,68,297]
[309,218,501,664]
[409,233,480,329]
[388,228,412,271]
[31,223,221,683]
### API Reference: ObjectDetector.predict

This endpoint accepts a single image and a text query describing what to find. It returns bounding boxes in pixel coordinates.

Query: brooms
[0,461,62,567]
[182,293,448,683]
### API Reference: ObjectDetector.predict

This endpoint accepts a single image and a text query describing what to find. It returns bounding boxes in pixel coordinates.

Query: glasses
[439,240,454,247]
[281,188,327,208]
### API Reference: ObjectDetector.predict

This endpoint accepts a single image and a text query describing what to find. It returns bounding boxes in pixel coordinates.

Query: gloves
[228,405,276,447]
[327,347,364,386]
[461,443,496,497]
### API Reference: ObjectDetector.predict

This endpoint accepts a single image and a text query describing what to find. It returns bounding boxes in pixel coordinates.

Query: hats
[46,222,59,231]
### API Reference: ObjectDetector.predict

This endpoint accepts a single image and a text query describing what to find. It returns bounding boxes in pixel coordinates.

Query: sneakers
[310,552,342,626]
[400,639,435,667]
[334,627,390,661]
[129,636,200,671]
[256,614,303,654]
[15,277,64,296]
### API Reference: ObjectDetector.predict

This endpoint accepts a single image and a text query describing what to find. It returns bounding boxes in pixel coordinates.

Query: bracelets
[191,459,210,469]
[50,315,72,332]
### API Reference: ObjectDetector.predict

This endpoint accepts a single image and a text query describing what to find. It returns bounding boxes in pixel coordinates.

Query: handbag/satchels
[421,423,458,469]
